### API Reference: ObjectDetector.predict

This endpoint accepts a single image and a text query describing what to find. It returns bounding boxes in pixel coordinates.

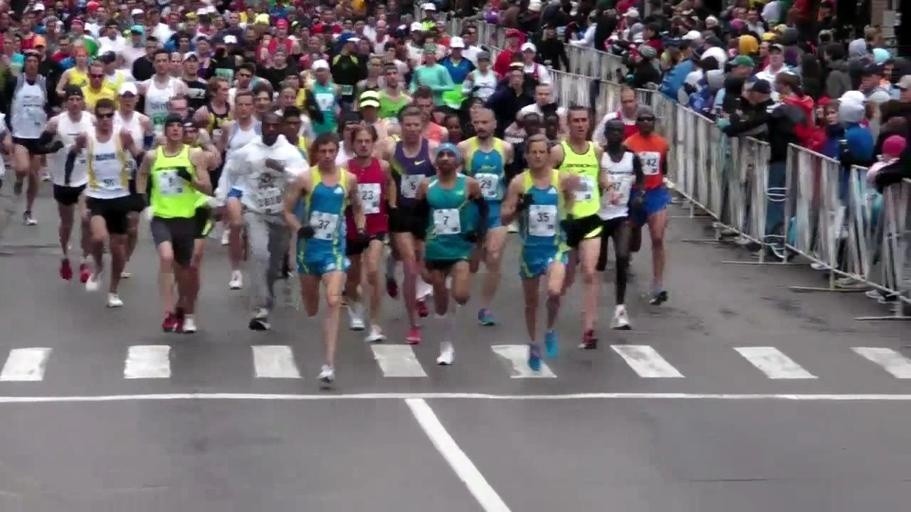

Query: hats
[856,62,884,76]
[747,80,771,94]
[623,8,639,18]
[24,0,238,130]
[726,54,757,68]
[277,2,537,166]
[768,43,784,54]
[680,29,702,41]
[837,99,865,123]
[882,135,907,159]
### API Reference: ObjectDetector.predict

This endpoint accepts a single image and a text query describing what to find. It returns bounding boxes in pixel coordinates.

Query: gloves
[177,166,191,180]
[297,224,314,238]
[517,194,532,213]
[356,231,369,247]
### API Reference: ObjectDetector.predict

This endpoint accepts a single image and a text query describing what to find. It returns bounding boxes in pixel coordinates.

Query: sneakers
[545,328,558,358]
[228,270,242,289]
[13,179,23,194]
[582,329,596,348]
[340,274,496,345]
[22,211,37,226]
[529,338,542,369]
[39,166,50,181]
[669,196,897,304]
[649,288,667,305]
[437,344,454,365]
[611,309,631,330]
[162,304,197,334]
[248,313,270,331]
[221,227,230,246]
[61,255,131,308]
[317,364,334,385]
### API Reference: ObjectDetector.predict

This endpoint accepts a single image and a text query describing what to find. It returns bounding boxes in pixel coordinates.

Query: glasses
[637,114,654,121]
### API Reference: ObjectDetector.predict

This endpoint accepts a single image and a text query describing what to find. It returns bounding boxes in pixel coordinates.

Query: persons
[2,0,911,390]
